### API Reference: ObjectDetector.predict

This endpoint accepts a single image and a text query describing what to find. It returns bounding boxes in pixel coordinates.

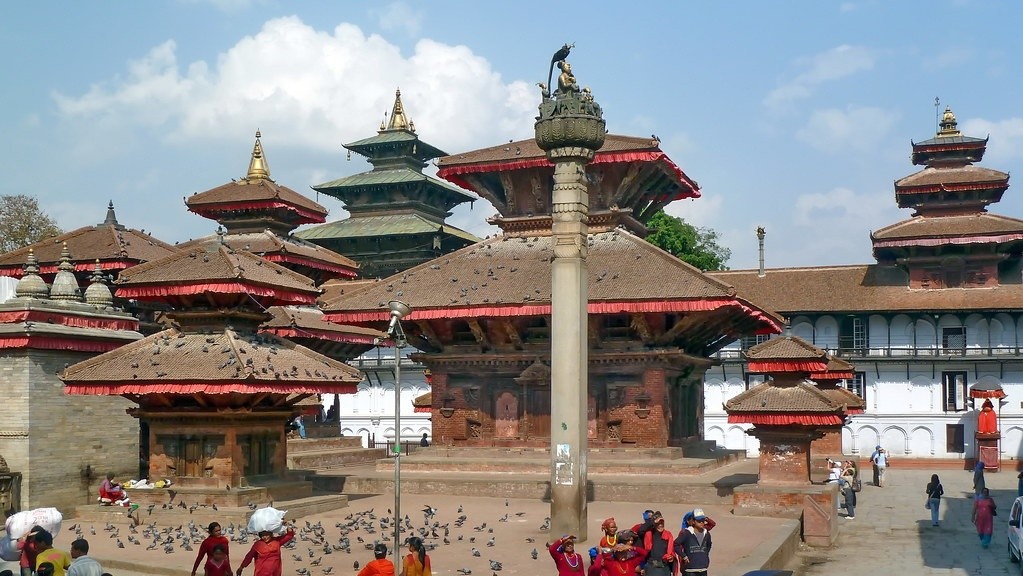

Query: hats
[692,508,706,520]
[834,461,842,467]
[562,535,574,544]
[876,446,881,449]
[651,511,665,523]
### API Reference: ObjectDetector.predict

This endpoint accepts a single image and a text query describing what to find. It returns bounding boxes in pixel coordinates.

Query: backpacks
[845,475,861,491]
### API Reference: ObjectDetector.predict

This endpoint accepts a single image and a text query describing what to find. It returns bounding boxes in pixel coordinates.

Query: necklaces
[564,552,578,568]
[618,561,628,573]
[607,534,616,546]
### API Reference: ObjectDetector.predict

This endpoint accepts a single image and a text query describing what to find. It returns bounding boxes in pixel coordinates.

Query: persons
[236,518,294,576]
[420,433,429,447]
[973,462,985,500]
[357,544,395,576]
[67,539,103,576]
[840,468,855,519]
[587,509,716,576]
[191,522,233,576]
[399,537,432,576]
[539,61,593,104]
[17,526,71,576]
[294,414,307,439]
[1018,472,1023,496]
[870,445,888,487]
[972,488,997,550]
[841,460,856,508]
[926,474,944,526]
[827,459,841,511]
[549,534,586,576]
[99,471,124,505]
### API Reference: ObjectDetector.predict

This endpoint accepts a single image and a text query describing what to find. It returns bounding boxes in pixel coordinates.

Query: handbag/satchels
[925,499,931,509]
[885,457,889,467]
[992,507,997,516]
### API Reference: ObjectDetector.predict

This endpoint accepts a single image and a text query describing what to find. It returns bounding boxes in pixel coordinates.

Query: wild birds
[459,129,661,160]
[194,176,671,307]
[69,480,550,576]
[51,229,210,284]
[762,399,768,408]
[35,297,359,379]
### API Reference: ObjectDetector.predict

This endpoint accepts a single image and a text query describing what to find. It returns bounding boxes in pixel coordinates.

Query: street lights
[385,299,413,576]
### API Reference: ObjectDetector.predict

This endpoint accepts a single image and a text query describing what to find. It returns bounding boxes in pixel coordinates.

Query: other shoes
[844,516,855,520]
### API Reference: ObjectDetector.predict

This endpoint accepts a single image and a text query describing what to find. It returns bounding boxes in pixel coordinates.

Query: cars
[1007,496,1023,567]
